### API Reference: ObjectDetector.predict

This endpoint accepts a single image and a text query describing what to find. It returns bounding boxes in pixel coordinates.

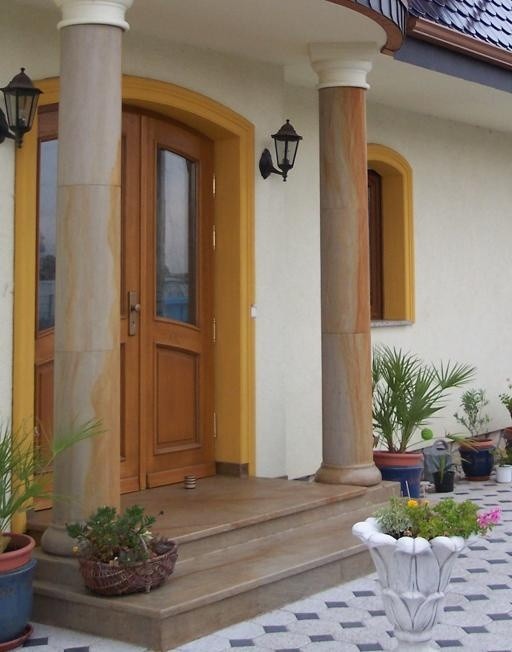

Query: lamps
[0,68,44,148]
[259,119,303,182]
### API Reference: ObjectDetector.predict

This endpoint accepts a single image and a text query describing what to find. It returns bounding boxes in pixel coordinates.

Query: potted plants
[66,503,178,595]
[371,345,512,499]
[0,414,107,651]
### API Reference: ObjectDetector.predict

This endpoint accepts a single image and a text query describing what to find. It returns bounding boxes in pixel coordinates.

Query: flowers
[374,498,501,541]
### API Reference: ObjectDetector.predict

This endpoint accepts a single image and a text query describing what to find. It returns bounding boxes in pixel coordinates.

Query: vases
[354,517,466,644]
